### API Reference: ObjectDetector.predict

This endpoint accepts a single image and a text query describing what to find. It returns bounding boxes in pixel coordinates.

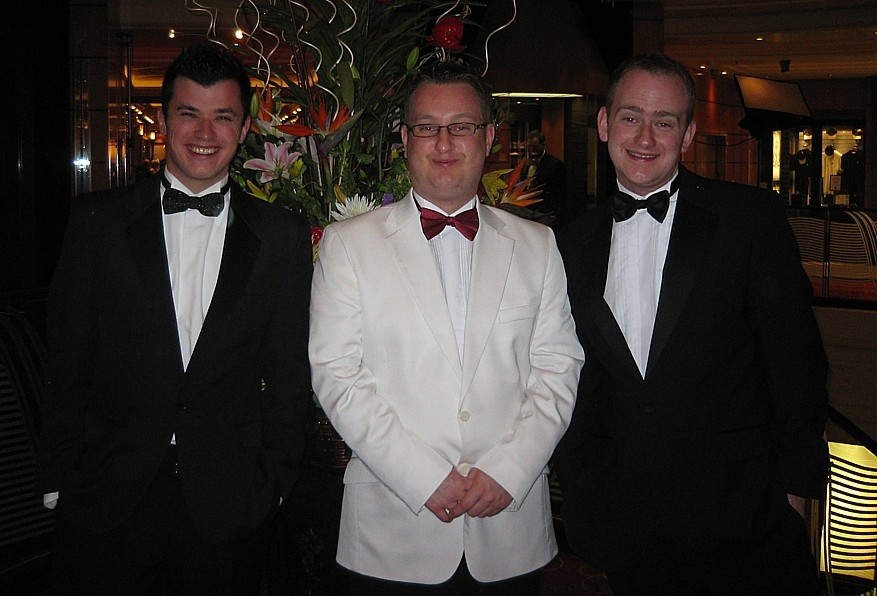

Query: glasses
[404,122,493,138]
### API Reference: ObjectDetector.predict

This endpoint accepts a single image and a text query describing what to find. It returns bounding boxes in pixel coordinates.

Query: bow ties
[160,165,231,217]
[412,195,480,242]
[609,173,682,224]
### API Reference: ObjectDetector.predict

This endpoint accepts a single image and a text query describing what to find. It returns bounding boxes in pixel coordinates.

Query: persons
[553,53,831,596]
[514,130,567,213]
[307,65,585,596]
[37,43,313,596]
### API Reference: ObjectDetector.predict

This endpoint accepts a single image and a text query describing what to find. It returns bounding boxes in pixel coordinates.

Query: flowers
[184,0,547,263]
